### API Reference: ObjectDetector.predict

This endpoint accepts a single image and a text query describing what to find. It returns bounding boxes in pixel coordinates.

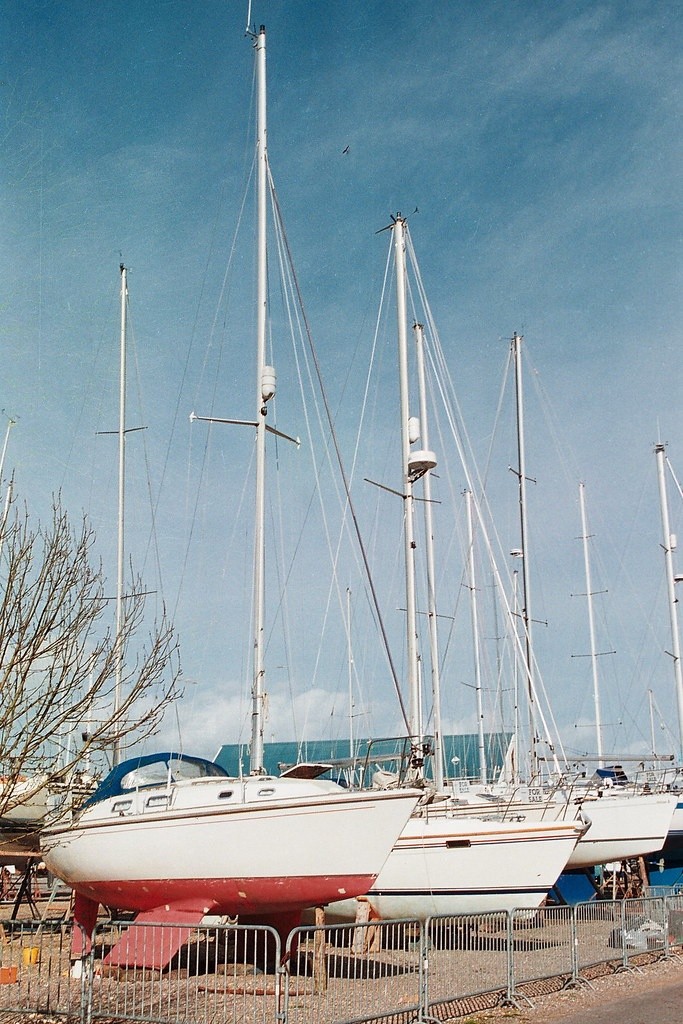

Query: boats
[0,652,124,836]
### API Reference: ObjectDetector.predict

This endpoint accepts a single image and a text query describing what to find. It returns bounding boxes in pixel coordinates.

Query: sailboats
[446,435,683,854]
[36,0,421,969]
[435,331,679,908]
[288,204,595,933]
